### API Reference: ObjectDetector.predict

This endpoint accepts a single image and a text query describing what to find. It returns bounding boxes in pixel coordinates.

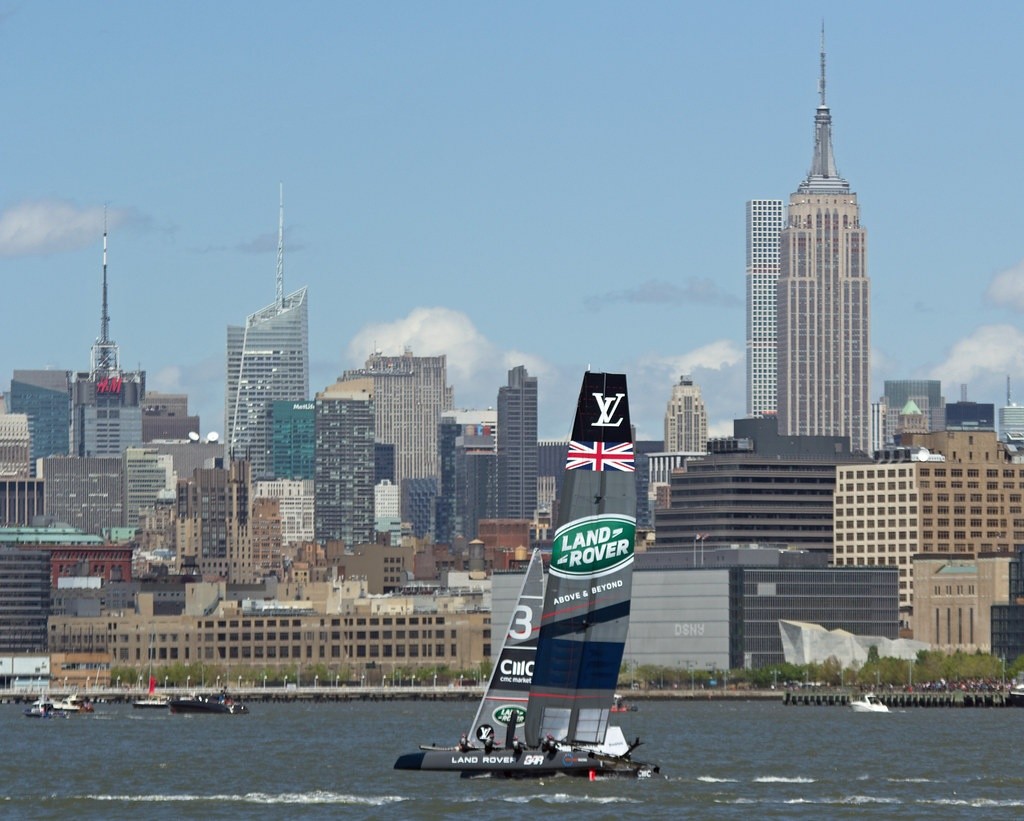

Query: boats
[134,698,170,708]
[169,694,249,714]
[611,693,637,711]
[1011,683,1024,705]
[849,692,891,714]
[26,690,70,719]
[51,696,91,711]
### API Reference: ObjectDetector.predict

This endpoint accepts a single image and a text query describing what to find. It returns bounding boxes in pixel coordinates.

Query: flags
[149,678,157,695]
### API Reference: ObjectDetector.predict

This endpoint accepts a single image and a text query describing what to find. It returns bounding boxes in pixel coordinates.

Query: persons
[218,689,227,704]
[546,734,560,753]
[485,734,500,754]
[512,737,528,754]
[459,733,476,752]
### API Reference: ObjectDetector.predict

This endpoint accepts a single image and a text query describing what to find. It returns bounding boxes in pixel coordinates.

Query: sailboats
[391,367,658,780]
